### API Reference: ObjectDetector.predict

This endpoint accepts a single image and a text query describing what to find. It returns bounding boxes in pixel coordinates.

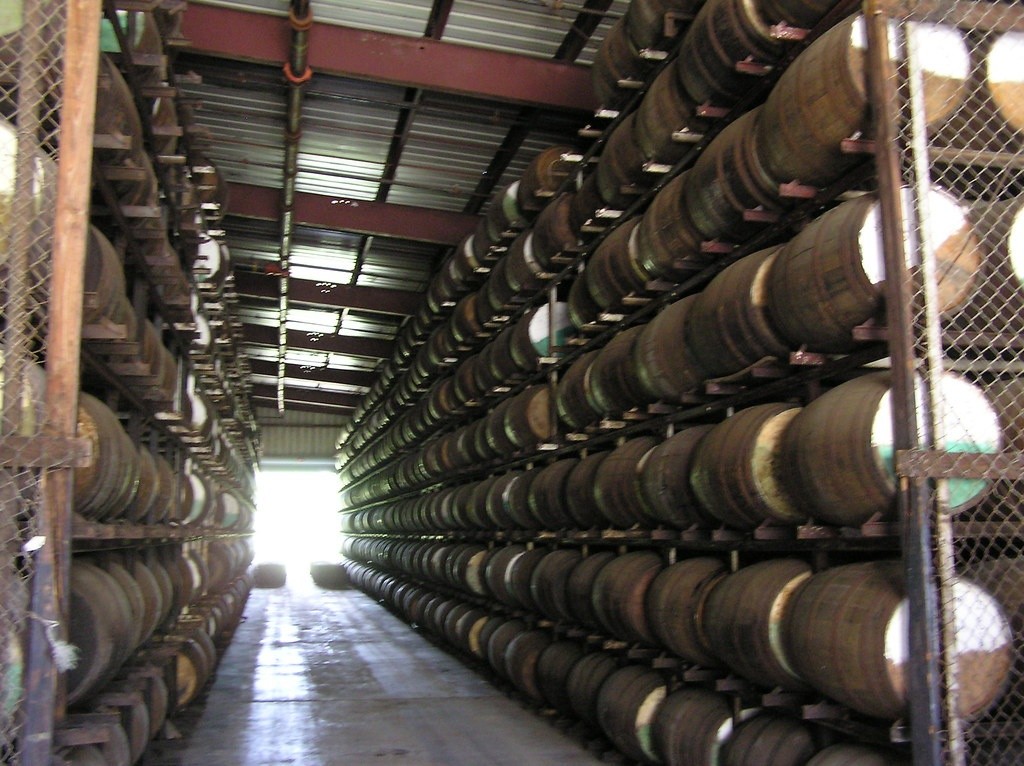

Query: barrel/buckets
[1,0,262,766]
[332,0,1024,766]
